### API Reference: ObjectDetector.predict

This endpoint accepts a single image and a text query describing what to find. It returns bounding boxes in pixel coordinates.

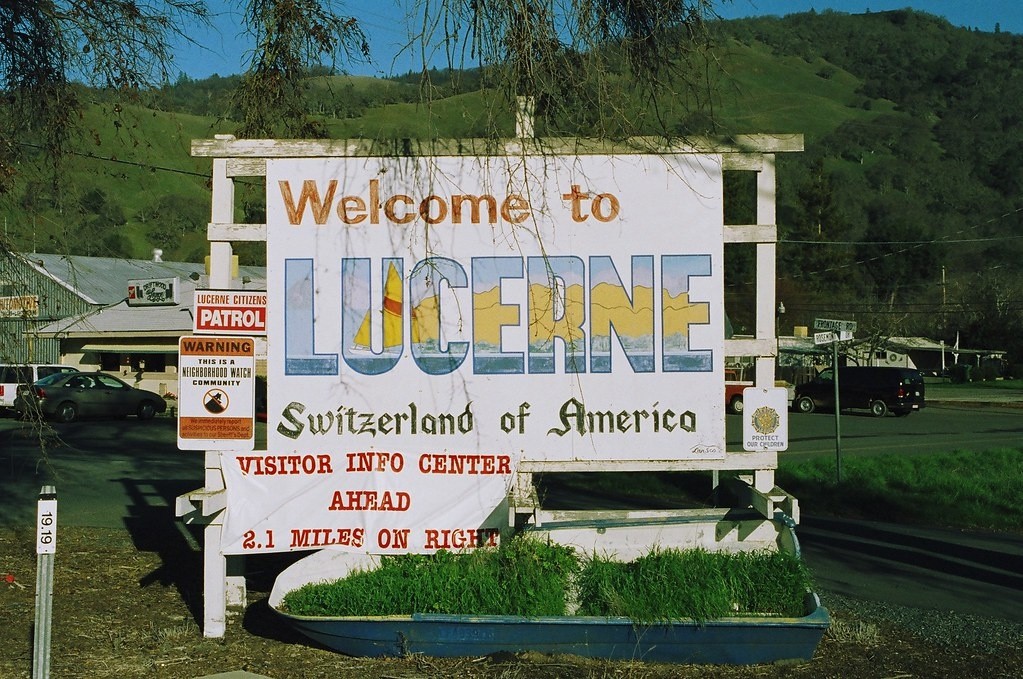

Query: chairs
[69,378,83,386]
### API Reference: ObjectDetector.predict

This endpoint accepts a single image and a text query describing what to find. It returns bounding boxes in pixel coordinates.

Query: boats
[268,508,834,668]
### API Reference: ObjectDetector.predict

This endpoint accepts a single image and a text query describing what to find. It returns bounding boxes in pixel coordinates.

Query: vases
[165,399,174,408]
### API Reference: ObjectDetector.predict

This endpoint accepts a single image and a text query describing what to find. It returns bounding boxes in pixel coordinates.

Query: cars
[14,371,167,423]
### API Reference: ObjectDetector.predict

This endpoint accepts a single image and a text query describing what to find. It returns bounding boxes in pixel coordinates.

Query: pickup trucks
[724,369,754,414]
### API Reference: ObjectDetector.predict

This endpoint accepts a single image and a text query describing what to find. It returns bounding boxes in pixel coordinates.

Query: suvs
[794,364,927,418]
[1,362,111,415]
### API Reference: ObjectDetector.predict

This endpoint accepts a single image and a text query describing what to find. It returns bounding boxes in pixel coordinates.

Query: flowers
[163,392,176,400]
